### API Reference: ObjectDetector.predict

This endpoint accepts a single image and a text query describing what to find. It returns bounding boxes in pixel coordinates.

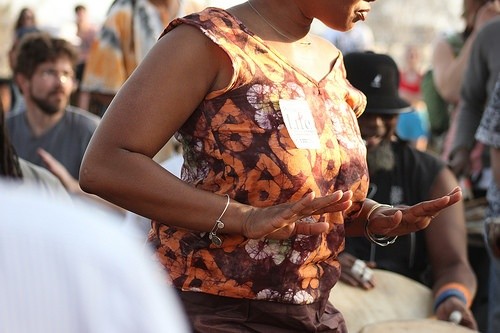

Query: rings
[430,215,435,218]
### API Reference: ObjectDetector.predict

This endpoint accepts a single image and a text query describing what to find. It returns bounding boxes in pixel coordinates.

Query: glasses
[35,68,74,84]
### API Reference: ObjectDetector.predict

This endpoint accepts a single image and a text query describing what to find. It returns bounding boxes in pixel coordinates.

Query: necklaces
[248,0,312,46]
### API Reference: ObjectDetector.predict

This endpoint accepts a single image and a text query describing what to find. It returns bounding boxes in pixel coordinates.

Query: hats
[343,51,412,113]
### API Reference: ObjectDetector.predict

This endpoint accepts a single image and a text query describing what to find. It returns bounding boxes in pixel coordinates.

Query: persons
[79,0,461,333]
[0,0,500,333]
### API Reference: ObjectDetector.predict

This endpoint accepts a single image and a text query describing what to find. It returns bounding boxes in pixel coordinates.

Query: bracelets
[210,194,230,247]
[365,204,399,247]
[433,290,467,311]
